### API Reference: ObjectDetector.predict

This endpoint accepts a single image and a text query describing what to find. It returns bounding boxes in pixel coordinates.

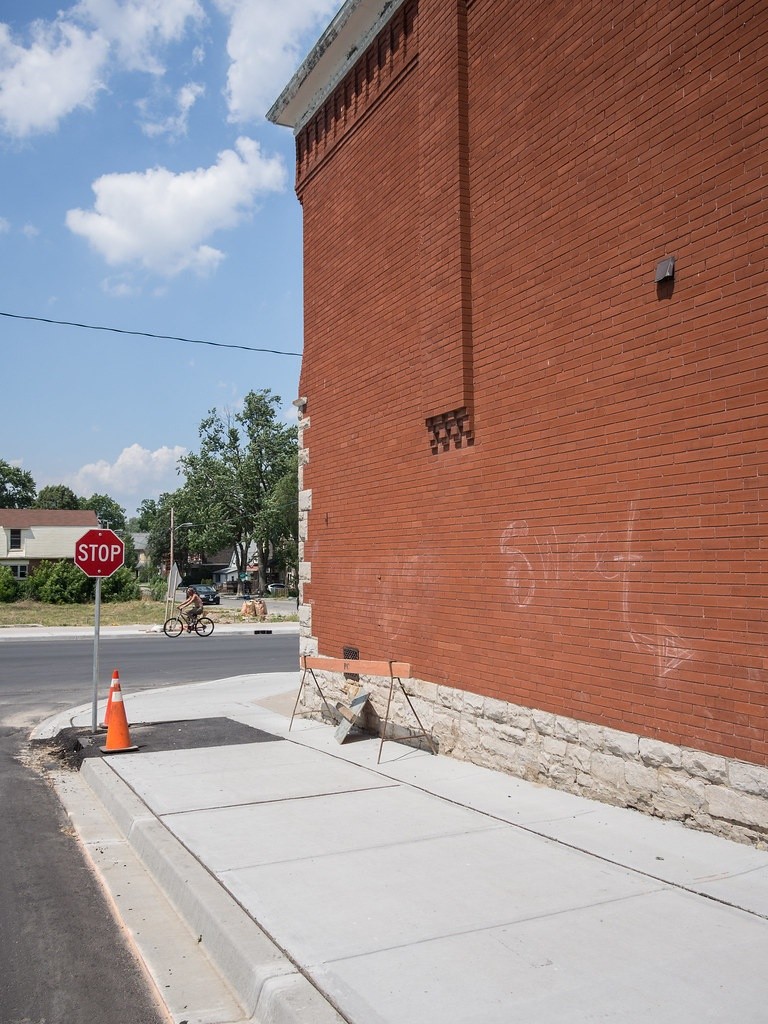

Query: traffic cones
[99,683,138,752]
[98,670,131,729]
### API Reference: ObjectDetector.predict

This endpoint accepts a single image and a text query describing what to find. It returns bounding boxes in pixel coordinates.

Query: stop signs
[74,529,125,577]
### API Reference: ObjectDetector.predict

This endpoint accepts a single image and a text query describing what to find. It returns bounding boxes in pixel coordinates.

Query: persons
[177,587,203,633]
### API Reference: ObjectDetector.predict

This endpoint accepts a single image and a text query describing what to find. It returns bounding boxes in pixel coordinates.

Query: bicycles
[163,606,214,638]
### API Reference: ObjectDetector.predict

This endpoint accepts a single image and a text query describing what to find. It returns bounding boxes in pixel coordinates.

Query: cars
[257,583,285,594]
[186,584,220,605]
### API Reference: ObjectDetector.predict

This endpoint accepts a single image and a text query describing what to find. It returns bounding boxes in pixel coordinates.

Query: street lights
[170,523,193,567]
[107,522,112,529]
